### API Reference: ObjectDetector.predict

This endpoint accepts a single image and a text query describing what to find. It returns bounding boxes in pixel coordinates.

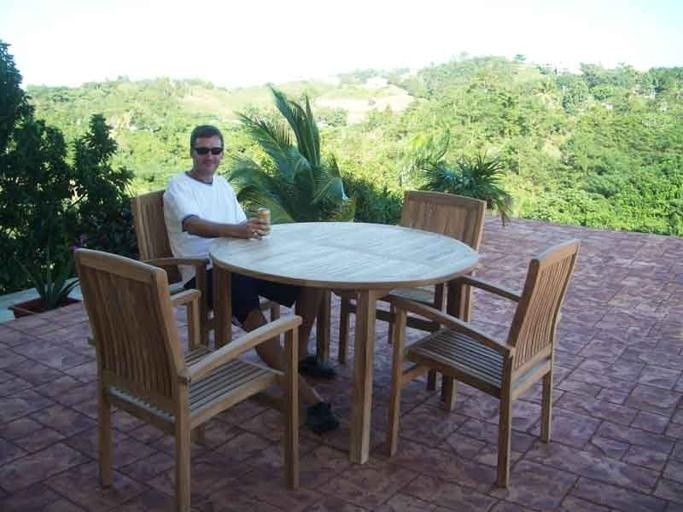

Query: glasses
[194,148,222,154]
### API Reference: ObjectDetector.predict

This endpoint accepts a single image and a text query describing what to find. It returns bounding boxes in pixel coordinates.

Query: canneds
[255,206,272,226]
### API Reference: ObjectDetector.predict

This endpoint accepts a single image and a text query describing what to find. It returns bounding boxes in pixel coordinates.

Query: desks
[209,221,479,465]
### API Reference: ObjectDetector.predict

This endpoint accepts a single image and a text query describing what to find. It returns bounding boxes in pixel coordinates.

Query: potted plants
[0,115,136,318]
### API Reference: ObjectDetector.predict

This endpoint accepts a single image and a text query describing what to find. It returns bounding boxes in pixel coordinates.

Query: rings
[251,229,259,236]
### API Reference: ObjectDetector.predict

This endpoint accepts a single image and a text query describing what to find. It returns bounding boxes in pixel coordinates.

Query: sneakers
[297,354,336,377]
[303,402,339,434]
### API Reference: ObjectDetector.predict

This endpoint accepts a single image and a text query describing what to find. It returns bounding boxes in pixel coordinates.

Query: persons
[163,124,339,435]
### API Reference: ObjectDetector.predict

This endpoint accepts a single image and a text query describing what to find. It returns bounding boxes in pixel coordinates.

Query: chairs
[133,189,281,347]
[386,238,581,489]
[73,248,303,512]
[338,191,487,391]
